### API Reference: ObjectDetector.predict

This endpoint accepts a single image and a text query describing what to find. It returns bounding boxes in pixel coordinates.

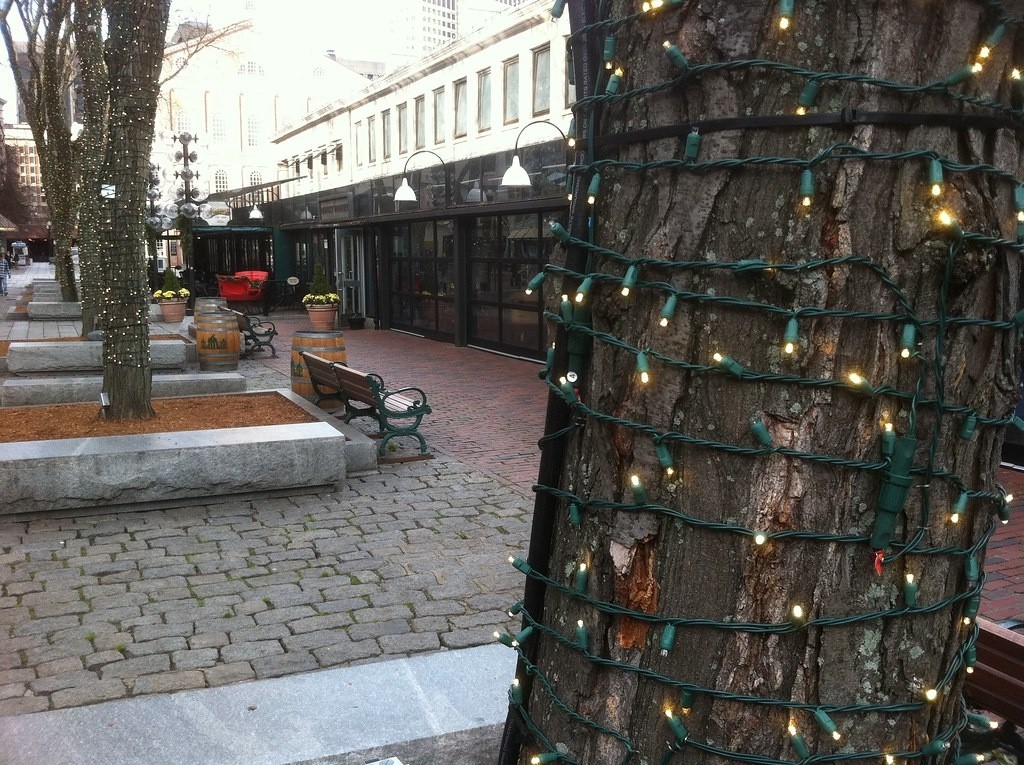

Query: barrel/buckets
[194,297,228,324]
[196,310,240,371]
[291,330,347,412]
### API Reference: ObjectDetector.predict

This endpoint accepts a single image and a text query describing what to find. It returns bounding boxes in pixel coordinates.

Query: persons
[5,251,11,269]
[0,253,12,297]
[510,263,519,286]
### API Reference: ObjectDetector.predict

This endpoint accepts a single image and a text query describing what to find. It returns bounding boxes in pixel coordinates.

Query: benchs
[214,306,278,357]
[300,349,431,458]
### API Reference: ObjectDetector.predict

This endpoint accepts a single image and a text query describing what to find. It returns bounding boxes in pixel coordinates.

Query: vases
[158,301,186,322]
[309,303,341,330]
[347,315,366,330]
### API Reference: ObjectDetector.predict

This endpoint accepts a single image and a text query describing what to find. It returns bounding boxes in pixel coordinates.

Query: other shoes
[5,291,8,296]
[0,292,3,296]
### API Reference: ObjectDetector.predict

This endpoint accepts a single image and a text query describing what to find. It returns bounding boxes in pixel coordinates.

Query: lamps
[247,190,281,223]
[394,151,454,204]
[498,120,569,189]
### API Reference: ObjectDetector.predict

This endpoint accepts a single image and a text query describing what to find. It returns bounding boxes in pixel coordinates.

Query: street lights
[162,132,212,313]
[143,161,164,294]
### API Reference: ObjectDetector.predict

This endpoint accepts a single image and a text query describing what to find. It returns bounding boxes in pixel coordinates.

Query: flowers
[152,288,193,301]
[301,293,342,306]
